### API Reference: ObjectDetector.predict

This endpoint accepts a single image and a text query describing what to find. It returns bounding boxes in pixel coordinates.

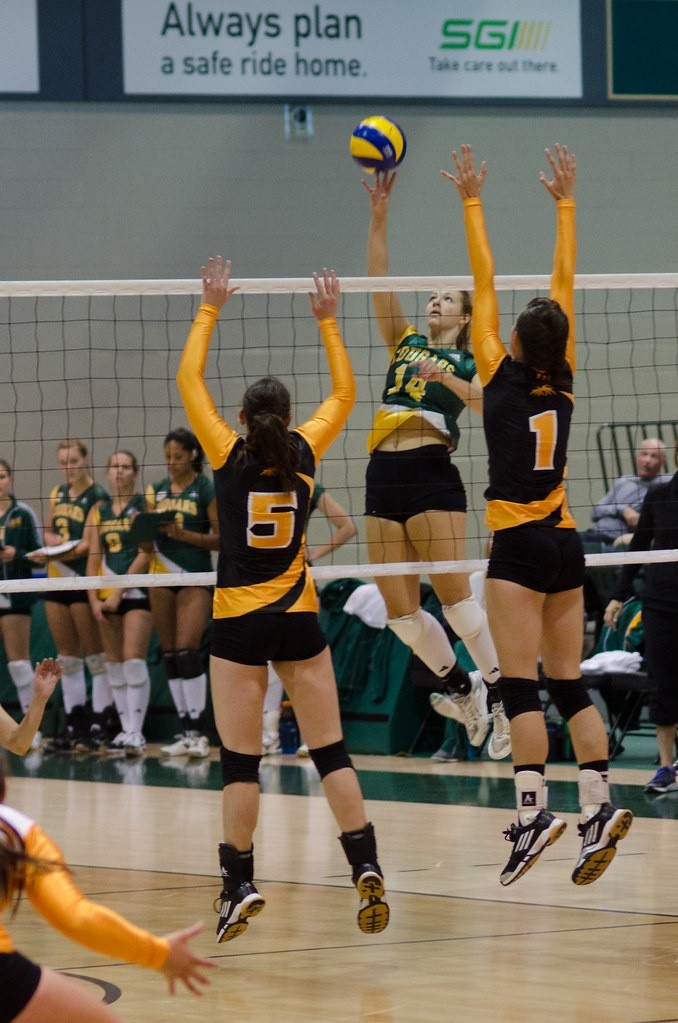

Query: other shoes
[431,747,462,762]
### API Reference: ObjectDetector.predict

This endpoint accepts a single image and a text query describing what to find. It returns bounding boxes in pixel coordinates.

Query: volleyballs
[349,117,409,174]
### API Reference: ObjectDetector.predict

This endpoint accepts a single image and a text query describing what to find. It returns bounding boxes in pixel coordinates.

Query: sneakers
[498,807,568,886]
[485,700,513,761]
[430,670,490,748]
[351,861,389,934]
[570,802,635,887]
[13,705,317,768]
[212,879,266,945]
[644,765,678,793]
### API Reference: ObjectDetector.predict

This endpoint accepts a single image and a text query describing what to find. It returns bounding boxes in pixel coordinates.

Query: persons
[0,759,220,1023]
[260,483,359,757]
[167,253,391,943]
[438,143,634,885]
[577,437,678,794]
[361,171,512,760]
[0,657,64,756]
[0,427,219,758]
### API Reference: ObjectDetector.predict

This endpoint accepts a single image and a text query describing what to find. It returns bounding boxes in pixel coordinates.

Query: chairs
[410,620,492,758]
[537,669,656,759]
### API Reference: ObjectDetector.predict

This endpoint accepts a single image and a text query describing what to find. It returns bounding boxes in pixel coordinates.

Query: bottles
[279,700,301,755]
